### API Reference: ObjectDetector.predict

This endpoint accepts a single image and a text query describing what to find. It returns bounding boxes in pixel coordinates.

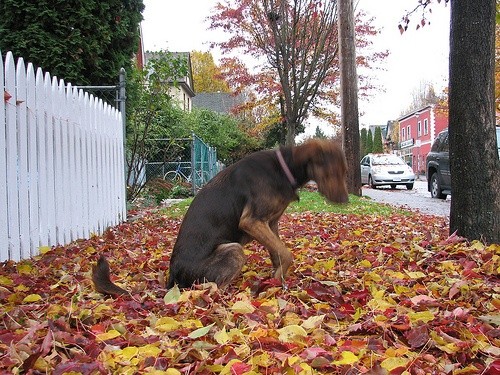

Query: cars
[360,153,415,191]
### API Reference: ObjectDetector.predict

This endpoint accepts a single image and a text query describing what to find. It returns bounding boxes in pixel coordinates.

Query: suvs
[425,126,500,200]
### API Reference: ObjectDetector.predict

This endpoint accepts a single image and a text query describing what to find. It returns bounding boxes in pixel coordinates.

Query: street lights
[416,112,421,181]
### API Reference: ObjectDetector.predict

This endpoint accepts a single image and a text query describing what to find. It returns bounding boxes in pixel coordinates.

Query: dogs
[89,139,349,302]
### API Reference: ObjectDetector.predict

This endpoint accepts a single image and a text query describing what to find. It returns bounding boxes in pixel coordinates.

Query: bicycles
[163,154,210,189]
